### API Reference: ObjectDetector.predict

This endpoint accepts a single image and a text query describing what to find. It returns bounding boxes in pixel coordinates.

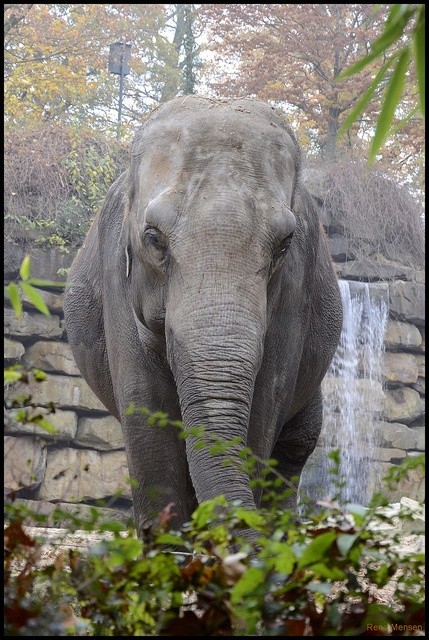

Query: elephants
[62,95,343,585]
